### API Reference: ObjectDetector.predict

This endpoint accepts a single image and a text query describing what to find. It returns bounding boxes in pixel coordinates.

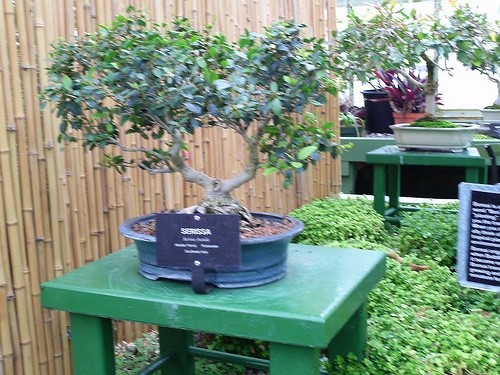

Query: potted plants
[332,0,500,151]
[38,6,353,289]
